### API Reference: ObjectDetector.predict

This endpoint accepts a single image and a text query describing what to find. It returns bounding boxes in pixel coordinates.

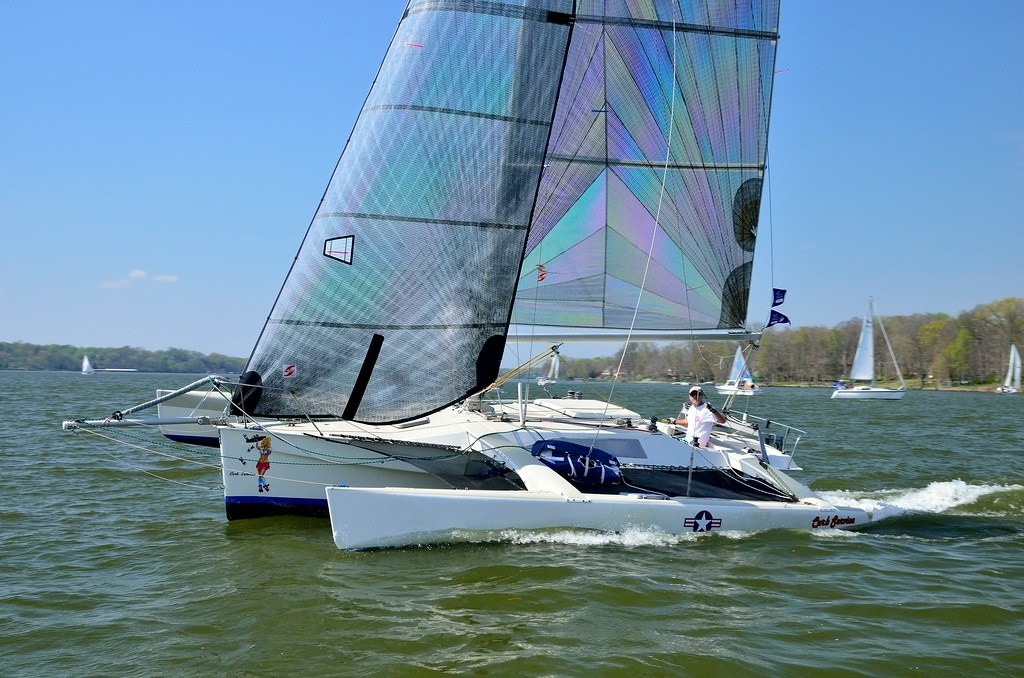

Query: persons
[838,383,854,390]
[668,386,725,447]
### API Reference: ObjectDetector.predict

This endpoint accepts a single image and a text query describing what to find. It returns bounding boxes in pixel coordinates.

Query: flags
[772,288,786,307]
[766,309,791,327]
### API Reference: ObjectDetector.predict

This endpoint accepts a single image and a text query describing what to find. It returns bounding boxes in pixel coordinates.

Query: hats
[688,385,708,399]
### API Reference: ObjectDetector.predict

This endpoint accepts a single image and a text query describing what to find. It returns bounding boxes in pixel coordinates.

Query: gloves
[668,417,676,425]
[705,403,717,414]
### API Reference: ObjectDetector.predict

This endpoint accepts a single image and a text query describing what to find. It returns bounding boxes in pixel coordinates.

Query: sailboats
[715,345,760,395]
[829,295,908,399]
[995,344,1022,394]
[536,355,560,387]
[61,0,869,549]
[80,355,95,375]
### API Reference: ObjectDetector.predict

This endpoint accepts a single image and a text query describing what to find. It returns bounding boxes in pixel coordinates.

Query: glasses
[690,392,702,397]
